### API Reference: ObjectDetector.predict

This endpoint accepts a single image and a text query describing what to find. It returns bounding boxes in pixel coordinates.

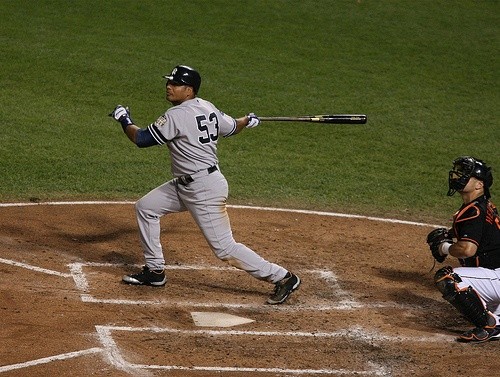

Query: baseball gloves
[426,227,453,263]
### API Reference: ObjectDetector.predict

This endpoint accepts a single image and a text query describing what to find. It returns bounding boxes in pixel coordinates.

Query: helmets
[163,64,201,96]
[473,162,493,198]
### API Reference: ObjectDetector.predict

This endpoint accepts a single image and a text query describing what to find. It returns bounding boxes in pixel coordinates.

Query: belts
[179,166,217,187]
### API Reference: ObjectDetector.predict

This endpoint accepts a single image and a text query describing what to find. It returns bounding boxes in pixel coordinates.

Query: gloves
[112,105,133,132]
[245,114,260,129]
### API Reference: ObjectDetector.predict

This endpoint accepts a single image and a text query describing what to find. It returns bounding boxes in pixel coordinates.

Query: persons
[426,156,500,343]
[108,65,301,304]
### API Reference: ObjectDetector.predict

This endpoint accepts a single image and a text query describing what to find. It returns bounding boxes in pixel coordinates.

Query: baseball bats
[257,114,367,124]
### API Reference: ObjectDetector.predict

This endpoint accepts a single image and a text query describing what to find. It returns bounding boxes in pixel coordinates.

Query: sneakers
[267,272,301,304]
[121,265,167,286]
[465,322,500,344]
